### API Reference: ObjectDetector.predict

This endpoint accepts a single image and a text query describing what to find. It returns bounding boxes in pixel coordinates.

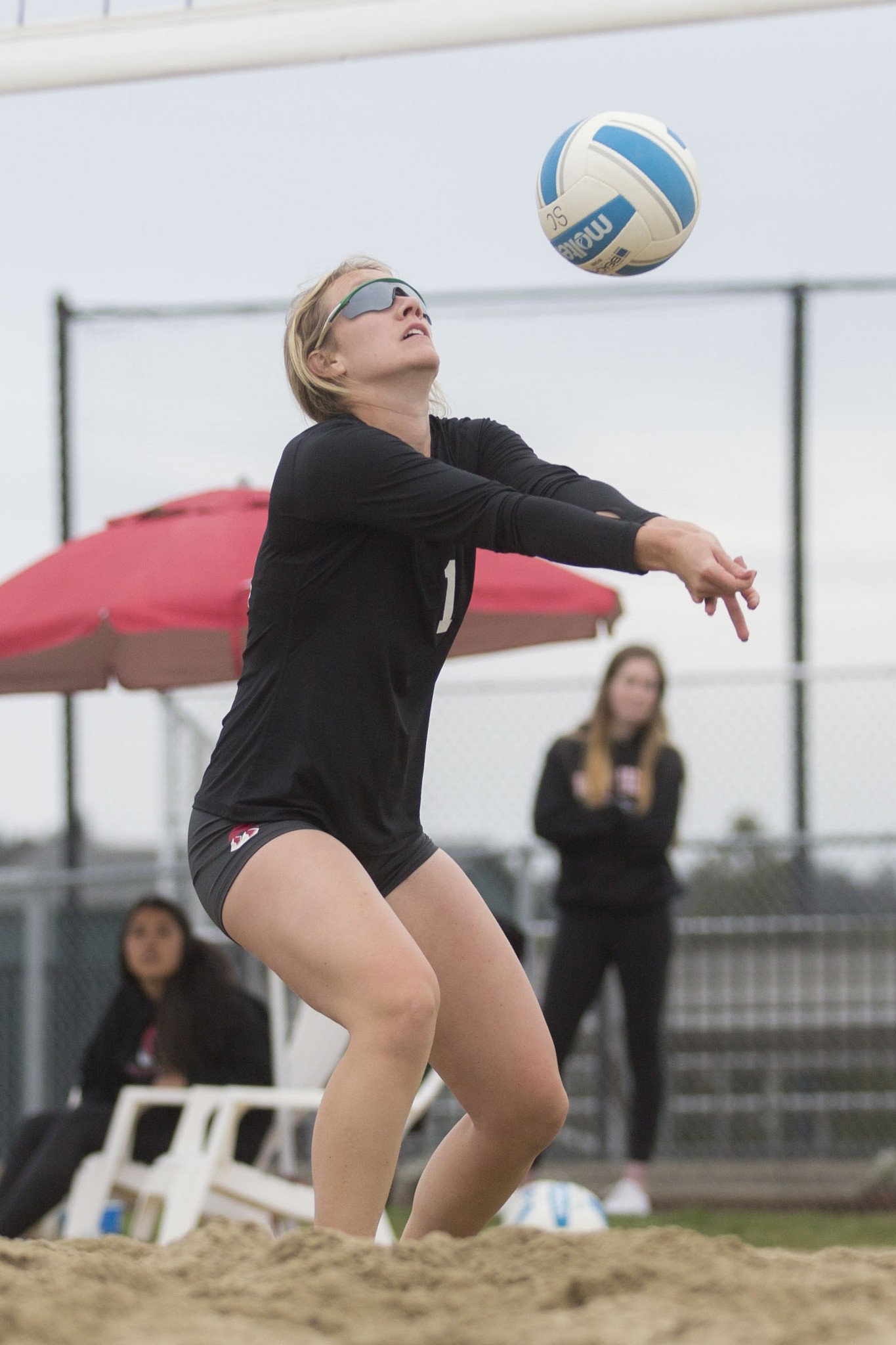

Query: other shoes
[602,1179,651,1219]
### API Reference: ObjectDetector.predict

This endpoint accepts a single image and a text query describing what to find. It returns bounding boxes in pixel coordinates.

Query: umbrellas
[0,471,618,1176]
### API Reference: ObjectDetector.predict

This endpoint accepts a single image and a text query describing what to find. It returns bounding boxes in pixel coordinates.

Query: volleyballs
[502,1179,607,1233]
[537,112,698,277]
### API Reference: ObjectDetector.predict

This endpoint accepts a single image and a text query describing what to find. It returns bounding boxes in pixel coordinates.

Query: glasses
[314,277,431,350]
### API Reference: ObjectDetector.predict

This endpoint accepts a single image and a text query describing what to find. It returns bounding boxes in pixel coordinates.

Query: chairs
[61,999,444,1256]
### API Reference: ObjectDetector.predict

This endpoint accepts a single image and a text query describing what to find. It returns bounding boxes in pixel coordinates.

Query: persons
[190,264,758,1241]
[524,650,688,1220]
[0,895,273,1239]
